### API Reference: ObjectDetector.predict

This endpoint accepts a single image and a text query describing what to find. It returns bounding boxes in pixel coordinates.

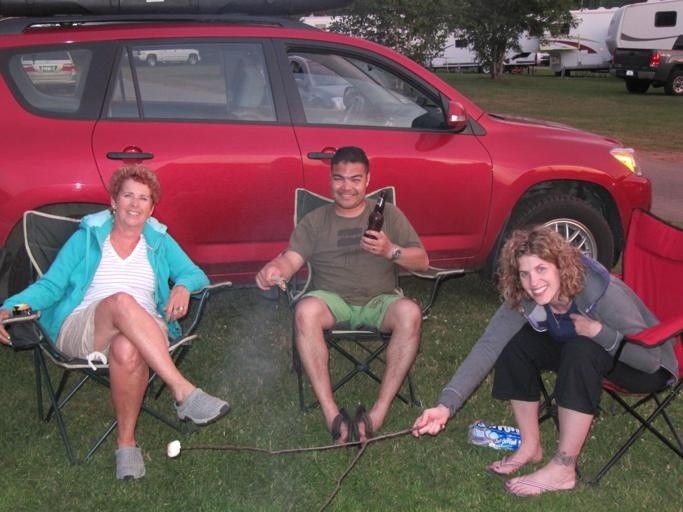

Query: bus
[297,13,542,76]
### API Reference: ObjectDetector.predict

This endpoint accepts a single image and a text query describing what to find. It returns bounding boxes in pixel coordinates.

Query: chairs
[290,189,463,410]
[222,51,270,123]
[0,208,237,476]
[525,209,682,488]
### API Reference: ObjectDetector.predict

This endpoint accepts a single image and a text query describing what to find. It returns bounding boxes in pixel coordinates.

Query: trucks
[300,14,549,73]
[0,0,651,308]
[605,1,683,56]
[539,7,617,76]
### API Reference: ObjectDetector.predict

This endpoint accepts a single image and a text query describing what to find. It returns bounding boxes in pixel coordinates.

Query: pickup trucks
[611,34,683,95]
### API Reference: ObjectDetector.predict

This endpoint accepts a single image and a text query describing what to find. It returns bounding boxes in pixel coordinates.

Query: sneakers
[115,443,146,480]
[174,388,230,425]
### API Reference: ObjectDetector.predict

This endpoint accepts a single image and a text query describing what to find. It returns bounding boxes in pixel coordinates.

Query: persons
[254,146,429,450]
[410,224,678,497]
[0,163,229,480]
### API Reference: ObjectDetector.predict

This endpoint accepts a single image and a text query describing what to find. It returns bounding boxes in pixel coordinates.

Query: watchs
[387,245,401,263]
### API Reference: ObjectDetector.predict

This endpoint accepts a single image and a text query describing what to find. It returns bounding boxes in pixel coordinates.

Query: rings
[175,306,182,312]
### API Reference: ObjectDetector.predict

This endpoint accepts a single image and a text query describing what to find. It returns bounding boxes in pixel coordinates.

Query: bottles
[363,188,388,239]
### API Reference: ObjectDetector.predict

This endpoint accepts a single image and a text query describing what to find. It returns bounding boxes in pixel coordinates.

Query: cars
[22,49,77,91]
[284,55,351,112]
[125,48,200,66]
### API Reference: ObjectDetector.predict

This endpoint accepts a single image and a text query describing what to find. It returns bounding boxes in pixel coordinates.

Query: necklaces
[547,299,572,329]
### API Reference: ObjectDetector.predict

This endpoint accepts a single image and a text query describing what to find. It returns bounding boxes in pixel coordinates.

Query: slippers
[332,406,373,443]
[485,456,526,474]
[505,472,576,496]
